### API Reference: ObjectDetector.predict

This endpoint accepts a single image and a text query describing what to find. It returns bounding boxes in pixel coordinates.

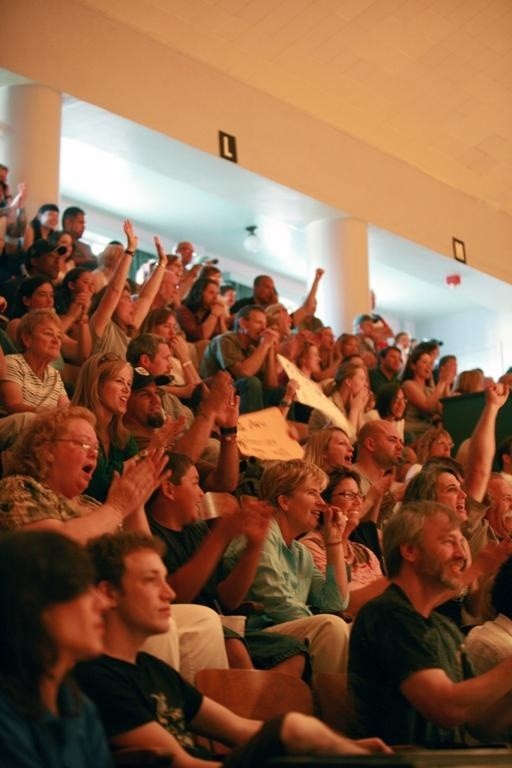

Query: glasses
[40,437,103,456]
[327,490,367,503]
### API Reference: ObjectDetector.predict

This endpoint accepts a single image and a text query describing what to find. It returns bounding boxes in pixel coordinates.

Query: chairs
[198,488,242,521]
[187,667,315,757]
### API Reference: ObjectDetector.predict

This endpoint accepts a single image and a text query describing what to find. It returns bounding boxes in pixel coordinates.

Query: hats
[25,238,67,261]
[128,363,172,393]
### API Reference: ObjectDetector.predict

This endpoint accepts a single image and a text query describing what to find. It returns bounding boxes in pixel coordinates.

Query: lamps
[244,226,258,250]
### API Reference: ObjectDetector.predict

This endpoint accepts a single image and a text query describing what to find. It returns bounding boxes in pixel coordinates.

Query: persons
[1,161,511,768]
